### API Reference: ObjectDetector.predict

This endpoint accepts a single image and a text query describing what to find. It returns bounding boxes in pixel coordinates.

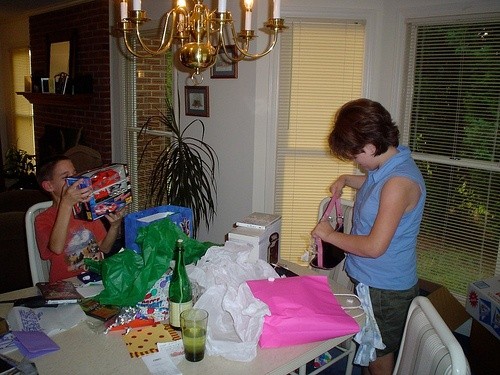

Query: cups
[179,309,208,361]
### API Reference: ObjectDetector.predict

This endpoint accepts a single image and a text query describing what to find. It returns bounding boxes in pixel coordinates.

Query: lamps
[121,0,287,84]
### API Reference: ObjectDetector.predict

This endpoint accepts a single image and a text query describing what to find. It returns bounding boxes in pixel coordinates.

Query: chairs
[25,201,56,286]
[393,296,470,375]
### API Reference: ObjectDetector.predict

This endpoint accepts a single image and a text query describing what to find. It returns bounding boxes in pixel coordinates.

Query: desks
[0,257,366,375]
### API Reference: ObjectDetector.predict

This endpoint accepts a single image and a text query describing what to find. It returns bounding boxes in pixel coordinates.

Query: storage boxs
[466,274,500,342]
[66,163,134,222]
[226,212,285,265]
[417,278,473,331]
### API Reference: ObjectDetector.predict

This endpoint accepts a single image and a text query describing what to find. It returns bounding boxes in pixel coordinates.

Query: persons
[34,155,124,290]
[310,98,427,375]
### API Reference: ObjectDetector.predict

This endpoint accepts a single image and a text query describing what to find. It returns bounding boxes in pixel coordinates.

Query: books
[37,280,77,305]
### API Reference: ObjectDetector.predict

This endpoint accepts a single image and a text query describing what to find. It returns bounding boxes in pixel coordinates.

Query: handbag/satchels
[246,276,366,349]
[310,192,345,269]
[124,203,193,260]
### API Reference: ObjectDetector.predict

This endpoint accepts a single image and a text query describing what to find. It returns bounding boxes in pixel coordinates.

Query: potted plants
[4,146,33,189]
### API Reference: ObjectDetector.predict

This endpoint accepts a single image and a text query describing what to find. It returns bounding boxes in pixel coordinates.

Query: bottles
[168,238,194,330]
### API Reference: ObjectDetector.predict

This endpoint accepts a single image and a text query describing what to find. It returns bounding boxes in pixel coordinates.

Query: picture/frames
[185,86,209,117]
[210,45,240,78]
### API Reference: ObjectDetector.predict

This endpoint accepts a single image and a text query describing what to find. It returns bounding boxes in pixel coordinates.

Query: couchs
[1,144,100,293]
